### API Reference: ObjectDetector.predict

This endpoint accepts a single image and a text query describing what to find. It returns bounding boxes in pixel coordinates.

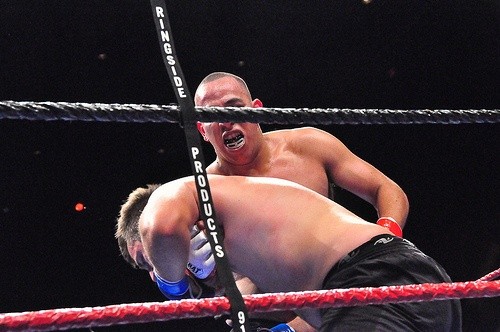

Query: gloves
[186,226,216,279]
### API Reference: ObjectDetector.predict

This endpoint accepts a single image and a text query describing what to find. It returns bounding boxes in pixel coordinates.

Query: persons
[185,72,410,332]
[114,175,463,332]
